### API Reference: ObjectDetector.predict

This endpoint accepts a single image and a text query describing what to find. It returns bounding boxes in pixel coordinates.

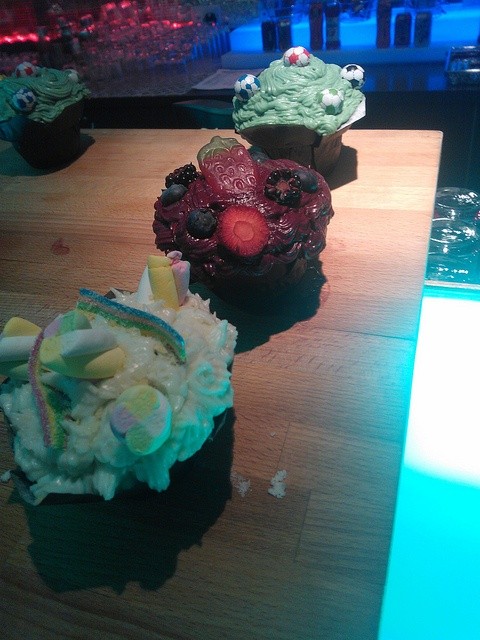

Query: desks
[0,121,444,640]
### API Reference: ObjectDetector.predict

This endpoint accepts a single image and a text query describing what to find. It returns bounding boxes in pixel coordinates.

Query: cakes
[0,61,92,172]
[152,137,334,316]
[0,251,239,506]
[232,46,367,172]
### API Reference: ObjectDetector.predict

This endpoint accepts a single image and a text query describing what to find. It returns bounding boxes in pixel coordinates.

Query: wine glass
[92,0,231,82]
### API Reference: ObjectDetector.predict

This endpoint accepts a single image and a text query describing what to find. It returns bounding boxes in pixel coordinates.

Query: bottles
[324,1,341,49]
[304,0,324,49]
[2,13,91,72]
[376,0,391,49]
